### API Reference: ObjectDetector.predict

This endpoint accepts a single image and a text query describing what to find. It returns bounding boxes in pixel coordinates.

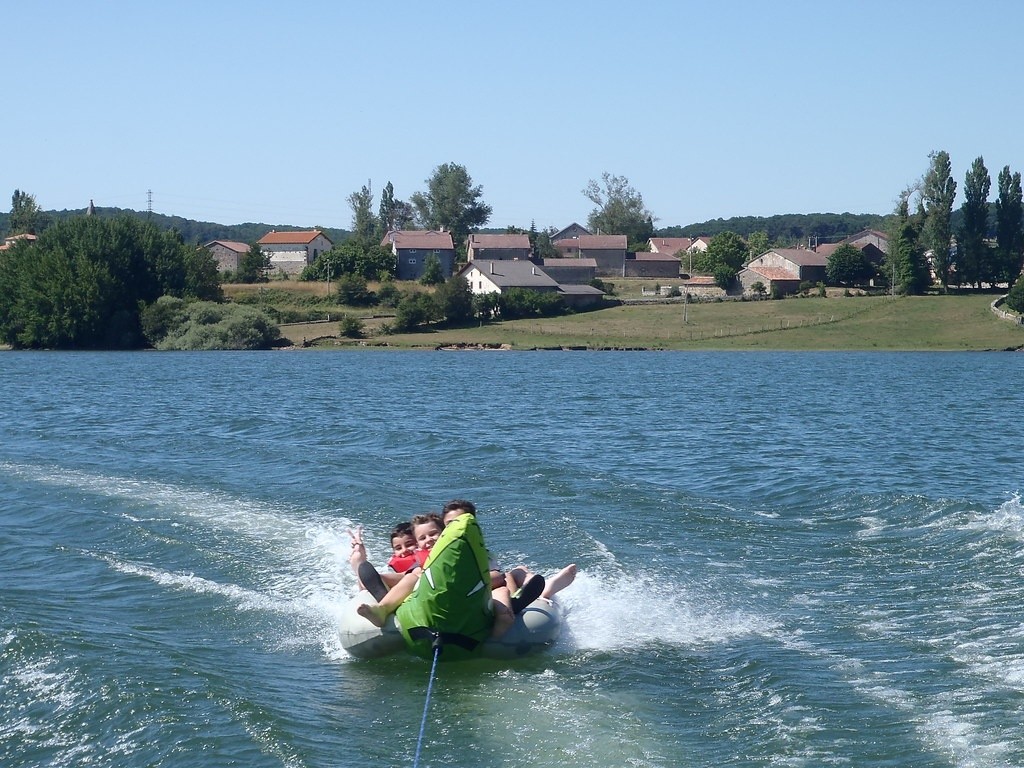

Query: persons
[345,499,578,637]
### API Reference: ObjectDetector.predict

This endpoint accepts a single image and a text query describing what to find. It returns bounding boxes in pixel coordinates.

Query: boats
[337,516,565,667]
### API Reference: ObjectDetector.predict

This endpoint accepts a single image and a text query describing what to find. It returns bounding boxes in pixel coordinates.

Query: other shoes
[358,561,391,603]
[511,574,545,615]
